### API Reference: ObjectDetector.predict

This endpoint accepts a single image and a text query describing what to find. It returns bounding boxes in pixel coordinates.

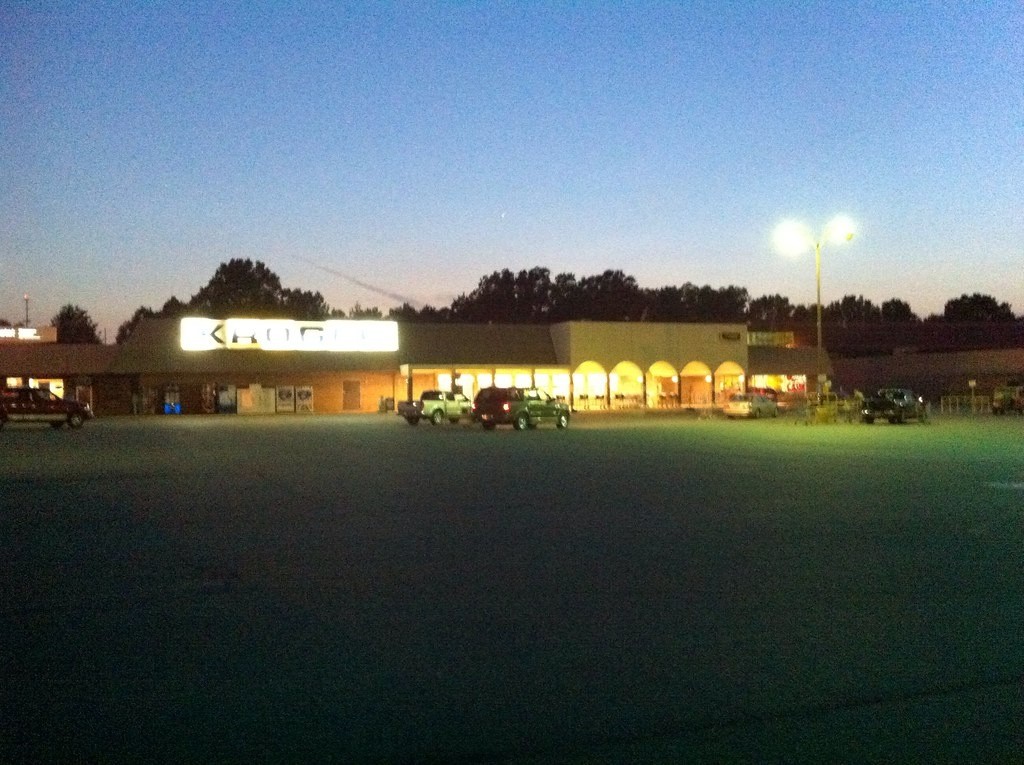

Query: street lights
[774,213,854,407]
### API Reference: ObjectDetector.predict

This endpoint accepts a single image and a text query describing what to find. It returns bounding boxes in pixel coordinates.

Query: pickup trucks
[475,385,572,431]
[0,386,95,429]
[397,390,473,427]
[861,388,932,424]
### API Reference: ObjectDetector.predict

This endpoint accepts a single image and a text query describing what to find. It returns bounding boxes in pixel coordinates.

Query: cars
[723,391,779,418]
